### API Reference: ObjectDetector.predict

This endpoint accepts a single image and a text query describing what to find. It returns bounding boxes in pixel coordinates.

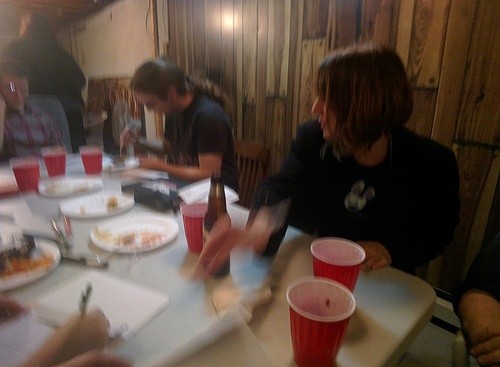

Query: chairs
[232,142,269,211]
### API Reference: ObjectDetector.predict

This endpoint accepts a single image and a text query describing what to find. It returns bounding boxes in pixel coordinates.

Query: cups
[180,203,208,256]
[79,145,103,176]
[285,279,357,367]
[40,146,66,178]
[310,239,366,293]
[10,157,40,193]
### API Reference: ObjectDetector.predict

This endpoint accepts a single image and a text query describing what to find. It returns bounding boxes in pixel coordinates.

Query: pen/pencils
[61,255,109,268]
[63,214,71,236]
[49,217,64,244]
[80,285,93,314]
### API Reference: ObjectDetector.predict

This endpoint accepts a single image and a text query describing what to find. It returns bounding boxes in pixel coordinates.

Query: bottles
[202,174,230,279]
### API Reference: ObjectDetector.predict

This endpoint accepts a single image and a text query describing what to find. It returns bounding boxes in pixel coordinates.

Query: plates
[103,159,140,172]
[89,216,179,254]
[38,179,102,198]
[0,242,62,293]
[59,193,136,218]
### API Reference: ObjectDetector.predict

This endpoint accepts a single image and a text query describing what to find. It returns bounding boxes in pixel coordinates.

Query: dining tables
[0,154,438,367]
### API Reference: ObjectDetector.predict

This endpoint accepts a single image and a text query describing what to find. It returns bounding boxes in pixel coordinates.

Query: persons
[129,59,240,192]
[452,233,500,367]
[194,44,461,277]
[0,62,72,163]
[6,14,88,154]
[0,293,133,367]
